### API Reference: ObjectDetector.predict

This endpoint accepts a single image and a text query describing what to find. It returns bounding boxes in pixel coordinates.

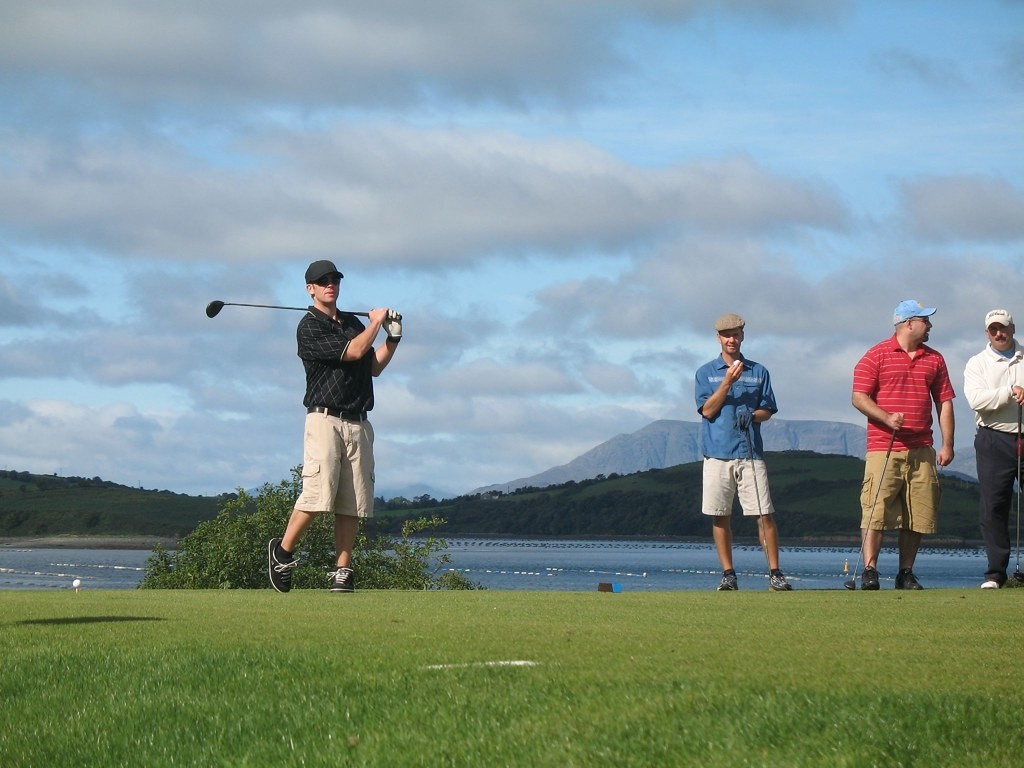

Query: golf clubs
[843,428,898,590]
[746,426,788,591]
[205,300,402,321]
[1013,402,1024,583]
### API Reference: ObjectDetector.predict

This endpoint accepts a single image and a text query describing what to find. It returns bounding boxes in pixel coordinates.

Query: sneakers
[327,566,355,592]
[268,537,300,593]
[980,581,999,589]
[769,572,792,591]
[895,568,923,590]
[717,574,738,591]
[861,564,881,590]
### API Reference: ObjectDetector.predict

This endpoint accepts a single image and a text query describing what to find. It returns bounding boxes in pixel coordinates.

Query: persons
[963,309,1024,588]
[269,260,402,593]
[852,299,956,590]
[694,314,792,592]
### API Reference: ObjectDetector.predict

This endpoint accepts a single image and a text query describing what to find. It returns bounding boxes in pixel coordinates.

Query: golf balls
[734,360,740,365]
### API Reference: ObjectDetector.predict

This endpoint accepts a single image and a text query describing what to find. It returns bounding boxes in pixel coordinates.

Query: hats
[305,260,344,283]
[893,300,937,326]
[715,313,745,331]
[985,309,1013,329]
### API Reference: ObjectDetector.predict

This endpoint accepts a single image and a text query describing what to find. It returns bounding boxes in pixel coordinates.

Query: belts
[307,406,367,421]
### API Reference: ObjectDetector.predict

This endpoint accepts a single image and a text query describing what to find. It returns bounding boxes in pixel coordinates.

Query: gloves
[382,309,402,337]
[733,410,756,428]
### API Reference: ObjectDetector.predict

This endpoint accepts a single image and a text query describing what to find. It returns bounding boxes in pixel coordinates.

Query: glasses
[911,317,929,324]
[317,276,340,285]
